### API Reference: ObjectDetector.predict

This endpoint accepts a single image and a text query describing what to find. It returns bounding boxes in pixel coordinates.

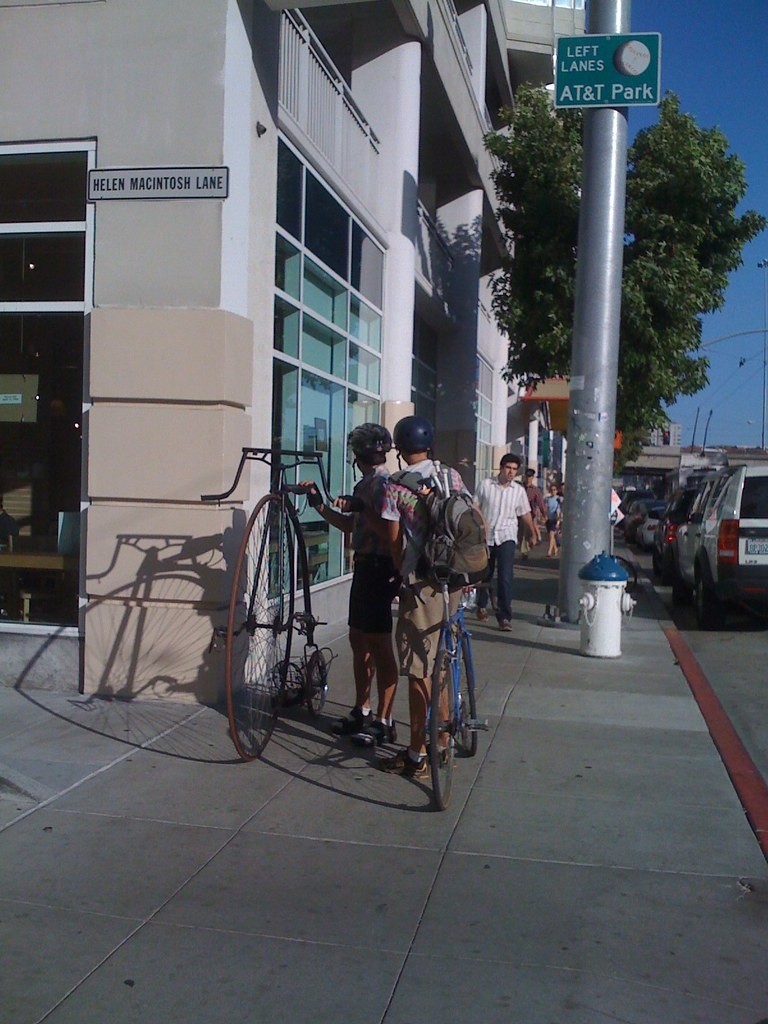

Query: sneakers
[332,707,374,734]
[379,751,429,780]
[351,719,397,747]
[427,744,448,769]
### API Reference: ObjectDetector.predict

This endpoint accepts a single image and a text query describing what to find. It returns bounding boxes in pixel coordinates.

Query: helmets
[393,416,433,453]
[350,423,391,453]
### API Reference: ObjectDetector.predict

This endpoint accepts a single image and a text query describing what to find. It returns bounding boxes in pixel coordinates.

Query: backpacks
[389,472,491,592]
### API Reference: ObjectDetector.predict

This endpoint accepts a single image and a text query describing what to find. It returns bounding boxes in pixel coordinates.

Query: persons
[381,416,475,779]
[299,423,397,746]
[544,485,561,557]
[557,483,565,496]
[0,495,20,553]
[521,470,547,558]
[473,454,537,631]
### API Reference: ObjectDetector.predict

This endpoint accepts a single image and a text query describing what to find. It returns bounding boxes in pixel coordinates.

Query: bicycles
[199,445,343,762]
[423,580,492,811]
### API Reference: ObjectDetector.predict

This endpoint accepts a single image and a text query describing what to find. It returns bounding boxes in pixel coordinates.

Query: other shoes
[499,619,512,631]
[478,606,487,621]
[547,549,551,558]
[554,548,558,557]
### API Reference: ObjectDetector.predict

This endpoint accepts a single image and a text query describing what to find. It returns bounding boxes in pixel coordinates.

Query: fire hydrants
[577,550,637,662]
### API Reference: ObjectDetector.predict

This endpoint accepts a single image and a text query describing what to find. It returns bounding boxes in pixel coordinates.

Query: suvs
[619,459,768,632]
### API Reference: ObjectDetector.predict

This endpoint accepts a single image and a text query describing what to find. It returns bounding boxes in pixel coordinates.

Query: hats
[525,469,535,476]
